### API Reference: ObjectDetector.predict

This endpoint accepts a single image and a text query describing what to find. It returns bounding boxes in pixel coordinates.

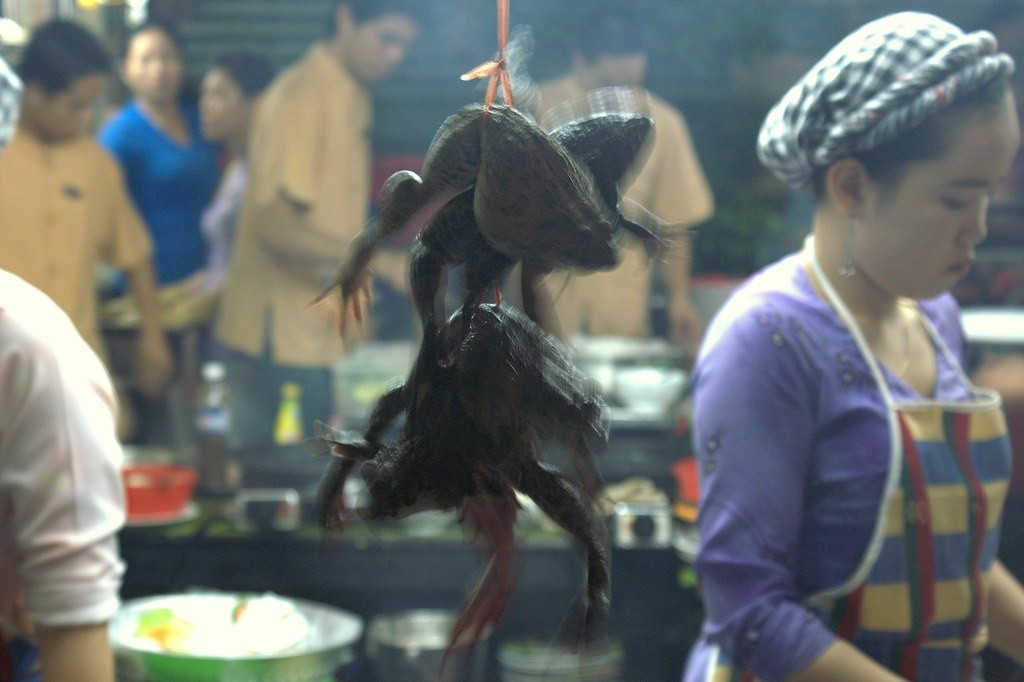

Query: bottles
[195,363,233,496]
[274,382,304,445]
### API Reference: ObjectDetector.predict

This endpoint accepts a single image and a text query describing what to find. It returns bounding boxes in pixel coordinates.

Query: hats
[756,12,1013,191]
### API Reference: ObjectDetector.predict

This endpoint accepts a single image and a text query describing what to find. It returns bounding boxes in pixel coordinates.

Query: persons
[678,9,1024,682]
[496,0,718,374]
[0,1,413,495]
[0,268,129,682]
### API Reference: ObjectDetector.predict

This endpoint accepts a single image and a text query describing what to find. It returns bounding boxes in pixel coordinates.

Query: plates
[126,503,201,526]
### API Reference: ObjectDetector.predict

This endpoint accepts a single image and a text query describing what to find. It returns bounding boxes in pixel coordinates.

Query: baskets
[121,464,194,516]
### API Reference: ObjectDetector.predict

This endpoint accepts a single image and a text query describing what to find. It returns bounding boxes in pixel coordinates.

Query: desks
[117,336,705,682]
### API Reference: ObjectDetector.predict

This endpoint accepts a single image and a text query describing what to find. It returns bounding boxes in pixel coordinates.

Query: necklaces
[808,258,912,379]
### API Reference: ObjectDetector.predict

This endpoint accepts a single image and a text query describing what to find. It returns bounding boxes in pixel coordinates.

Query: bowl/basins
[613,370,689,413]
[108,593,362,682]
[123,466,196,511]
